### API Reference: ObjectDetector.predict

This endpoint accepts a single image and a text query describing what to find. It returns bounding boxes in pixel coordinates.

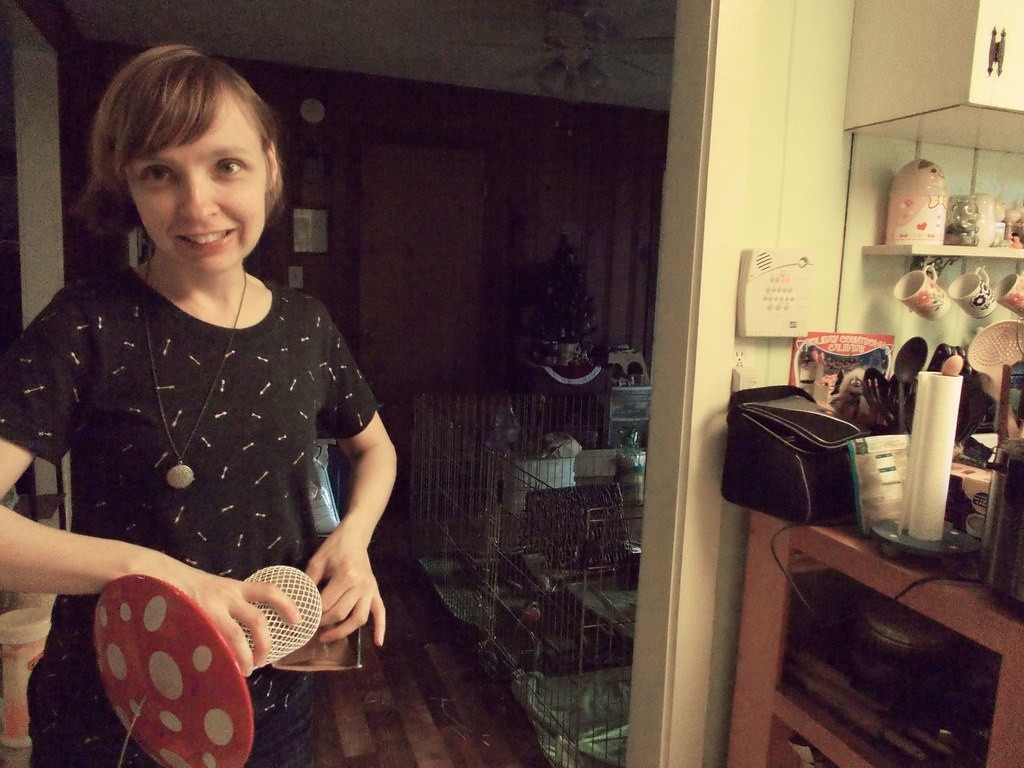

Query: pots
[977,437,1024,606]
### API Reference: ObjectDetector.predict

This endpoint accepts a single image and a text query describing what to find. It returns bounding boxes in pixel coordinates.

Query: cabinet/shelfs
[726,509,1024,768]
[840,0,1024,145]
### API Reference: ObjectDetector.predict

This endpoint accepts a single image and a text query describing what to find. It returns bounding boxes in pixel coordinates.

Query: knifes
[927,343,988,442]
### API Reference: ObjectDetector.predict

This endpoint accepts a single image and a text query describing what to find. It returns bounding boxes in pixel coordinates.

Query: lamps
[536,56,620,105]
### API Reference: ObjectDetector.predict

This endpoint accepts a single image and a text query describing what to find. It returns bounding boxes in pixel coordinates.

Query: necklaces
[144,253,247,489]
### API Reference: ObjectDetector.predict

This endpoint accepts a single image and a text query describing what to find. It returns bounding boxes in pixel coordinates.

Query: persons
[0,44,396,768]
[516,257,599,396]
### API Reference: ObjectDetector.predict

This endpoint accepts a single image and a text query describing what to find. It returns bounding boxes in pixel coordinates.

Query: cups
[995,270,1024,318]
[627,374,644,387]
[948,267,996,318]
[894,265,951,321]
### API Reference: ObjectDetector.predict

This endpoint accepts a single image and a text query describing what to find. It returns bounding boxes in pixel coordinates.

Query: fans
[442,0,677,78]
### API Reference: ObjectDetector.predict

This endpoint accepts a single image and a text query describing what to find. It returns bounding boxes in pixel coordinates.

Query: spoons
[894,336,928,435]
